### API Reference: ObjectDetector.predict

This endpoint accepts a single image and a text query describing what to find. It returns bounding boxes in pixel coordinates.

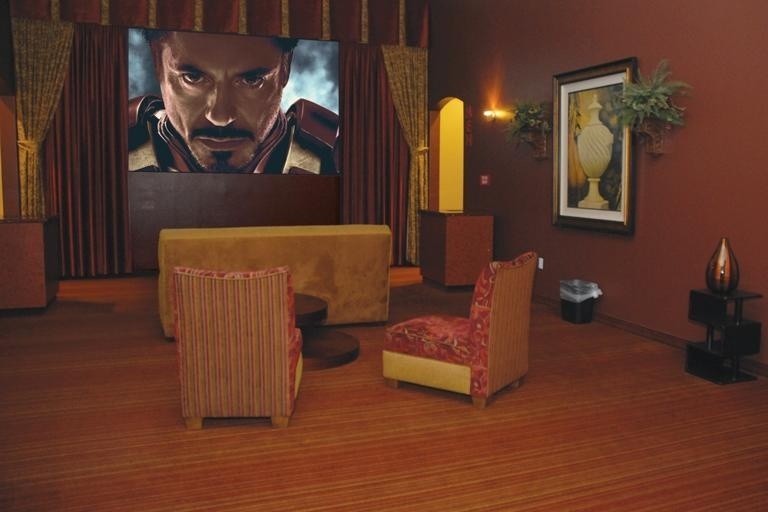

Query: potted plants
[614,60,695,156]
[500,97,551,159]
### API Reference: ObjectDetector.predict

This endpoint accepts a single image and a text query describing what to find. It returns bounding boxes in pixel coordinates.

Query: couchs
[155,222,392,340]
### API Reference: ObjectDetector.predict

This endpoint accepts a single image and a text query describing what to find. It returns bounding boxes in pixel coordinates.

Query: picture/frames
[551,57,635,235]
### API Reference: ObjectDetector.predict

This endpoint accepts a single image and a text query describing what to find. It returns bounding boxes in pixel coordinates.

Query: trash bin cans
[560,279,598,324]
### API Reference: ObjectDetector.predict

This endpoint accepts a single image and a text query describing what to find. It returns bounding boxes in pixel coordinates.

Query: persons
[127,28,341,177]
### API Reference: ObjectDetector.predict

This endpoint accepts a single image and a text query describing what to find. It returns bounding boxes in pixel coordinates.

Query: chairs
[172,264,304,429]
[382,253,539,407]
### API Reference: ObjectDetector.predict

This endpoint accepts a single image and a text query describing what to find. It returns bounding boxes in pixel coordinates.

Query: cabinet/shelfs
[684,287,768,386]
